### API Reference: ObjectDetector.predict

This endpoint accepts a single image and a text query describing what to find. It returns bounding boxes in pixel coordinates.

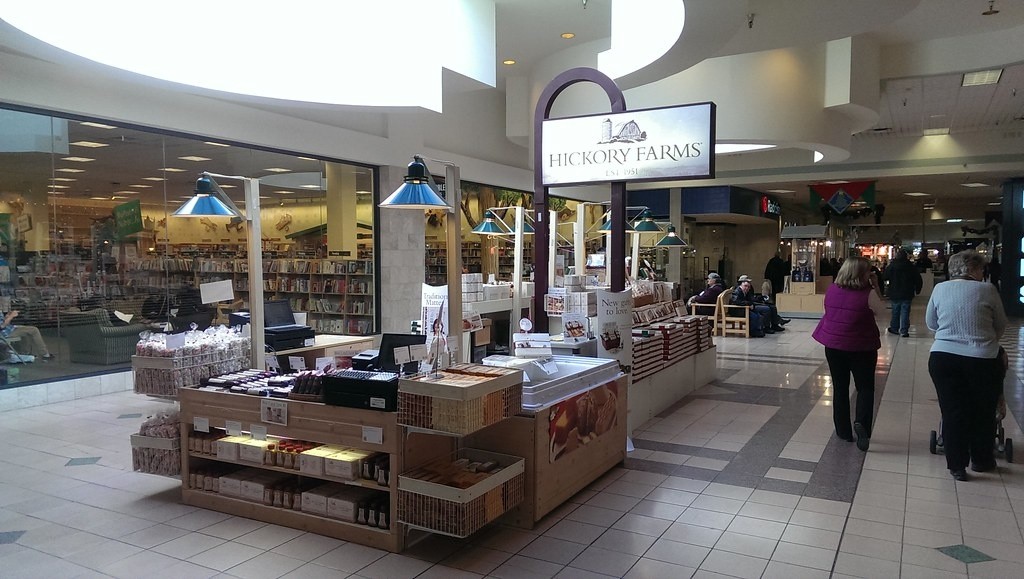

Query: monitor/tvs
[375,334,426,374]
[261,299,296,327]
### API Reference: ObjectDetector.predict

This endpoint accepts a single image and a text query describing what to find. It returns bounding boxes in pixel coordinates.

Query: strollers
[929,345,1013,463]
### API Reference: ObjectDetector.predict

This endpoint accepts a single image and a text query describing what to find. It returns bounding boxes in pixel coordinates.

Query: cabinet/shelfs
[178,384,455,555]
[122,254,374,335]
[426,234,601,284]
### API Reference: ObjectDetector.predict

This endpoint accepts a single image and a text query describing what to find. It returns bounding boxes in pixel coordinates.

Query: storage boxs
[299,485,327,517]
[299,445,347,475]
[327,487,365,519]
[324,448,375,478]
[218,473,240,498]
[239,478,268,502]
[216,434,241,463]
[240,438,276,461]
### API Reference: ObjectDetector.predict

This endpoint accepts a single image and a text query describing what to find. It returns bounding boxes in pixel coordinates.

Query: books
[0,247,481,336]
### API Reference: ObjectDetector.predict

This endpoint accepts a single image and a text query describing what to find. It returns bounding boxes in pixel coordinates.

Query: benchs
[721,287,750,340]
[689,289,735,339]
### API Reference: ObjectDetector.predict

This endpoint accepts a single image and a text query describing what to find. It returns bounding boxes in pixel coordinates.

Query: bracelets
[875,285,879,287]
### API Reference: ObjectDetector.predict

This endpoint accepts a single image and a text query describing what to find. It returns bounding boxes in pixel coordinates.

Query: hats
[738,275,752,282]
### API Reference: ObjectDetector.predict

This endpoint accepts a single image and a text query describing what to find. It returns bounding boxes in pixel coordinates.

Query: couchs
[109,296,147,325]
[57,299,149,366]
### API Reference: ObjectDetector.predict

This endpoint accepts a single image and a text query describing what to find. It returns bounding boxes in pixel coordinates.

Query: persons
[0,309,55,362]
[925,250,1008,480]
[812,256,887,452]
[687,251,791,337]
[425,318,448,366]
[821,248,999,338]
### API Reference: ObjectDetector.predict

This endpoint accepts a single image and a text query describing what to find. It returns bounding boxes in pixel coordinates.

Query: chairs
[0,332,27,366]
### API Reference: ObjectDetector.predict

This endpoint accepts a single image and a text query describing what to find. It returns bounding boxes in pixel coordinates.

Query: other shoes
[834,432,856,442]
[971,460,997,471]
[753,329,775,337]
[854,422,869,451]
[952,469,967,481]
[888,326,909,337]
[771,319,791,332]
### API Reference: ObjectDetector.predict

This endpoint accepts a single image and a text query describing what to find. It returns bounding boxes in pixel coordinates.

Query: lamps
[627,205,665,234]
[470,204,536,237]
[583,200,638,234]
[169,171,251,226]
[376,154,457,216]
[638,221,689,248]
